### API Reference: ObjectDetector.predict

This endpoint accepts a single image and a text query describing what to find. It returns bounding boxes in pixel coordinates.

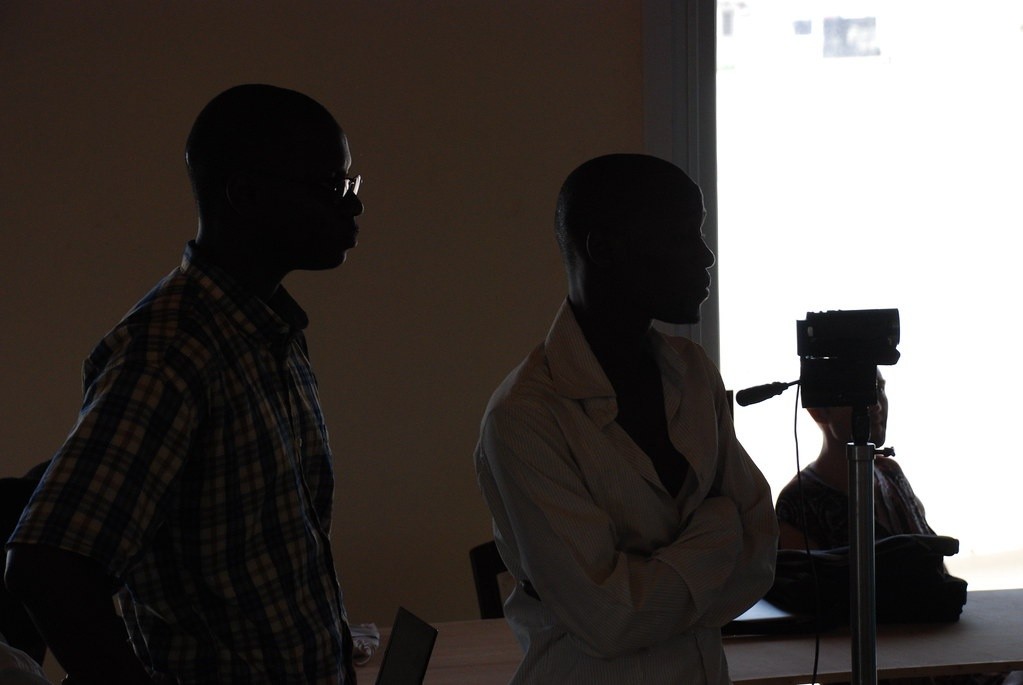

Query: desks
[352,586,1023,685]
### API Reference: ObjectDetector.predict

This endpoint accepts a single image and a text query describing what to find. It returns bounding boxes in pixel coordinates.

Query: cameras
[796,308,900,365]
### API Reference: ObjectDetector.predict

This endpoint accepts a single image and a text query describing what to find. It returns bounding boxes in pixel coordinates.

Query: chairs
[469,541,512,618]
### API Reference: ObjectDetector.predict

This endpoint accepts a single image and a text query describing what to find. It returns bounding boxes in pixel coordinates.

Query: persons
[0,83,367,685]
[775,369,944,575]
[473,152,780,685]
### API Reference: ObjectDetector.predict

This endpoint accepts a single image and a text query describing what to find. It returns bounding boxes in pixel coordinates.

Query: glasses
[267,165,363,200]
